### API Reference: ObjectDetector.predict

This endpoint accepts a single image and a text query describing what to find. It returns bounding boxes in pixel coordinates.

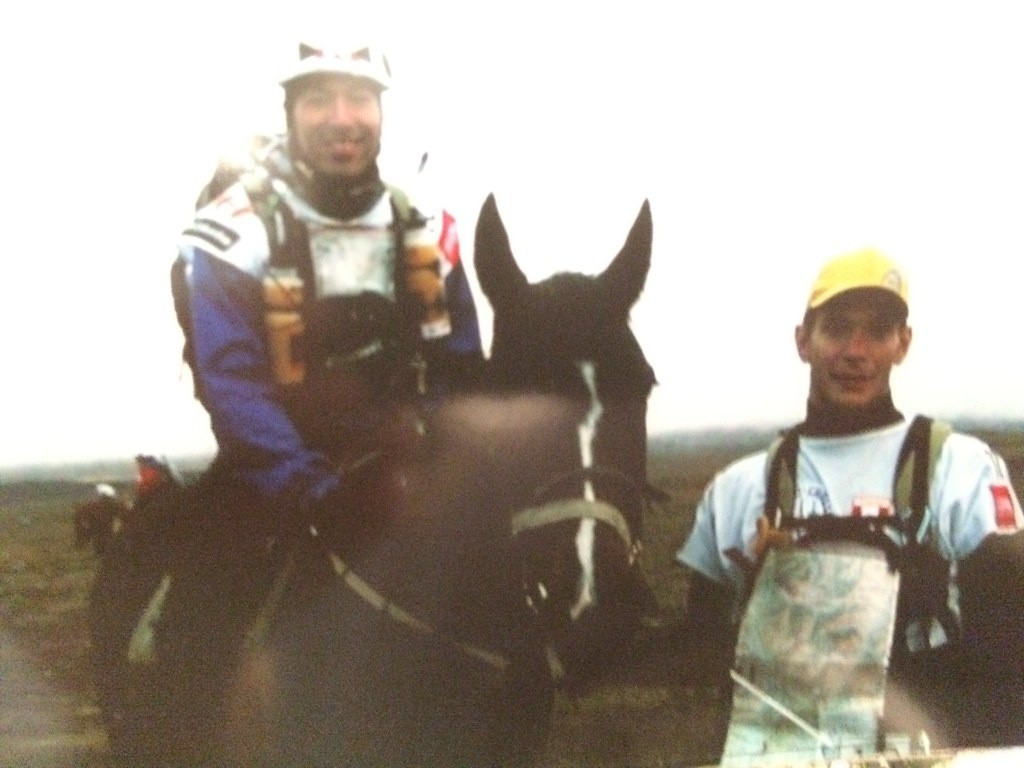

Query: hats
[278,31,392,91]
[808,251,910,321]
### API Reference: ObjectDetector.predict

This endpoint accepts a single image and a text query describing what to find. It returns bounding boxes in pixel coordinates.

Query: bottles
[259,242,307,388]
[402,205,454,340]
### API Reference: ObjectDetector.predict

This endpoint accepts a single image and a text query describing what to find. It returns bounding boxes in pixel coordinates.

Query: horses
[89,190,658,766]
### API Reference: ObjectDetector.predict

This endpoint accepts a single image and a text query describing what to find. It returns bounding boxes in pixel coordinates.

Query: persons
[670,249,1023,768]
[169,26,486,765]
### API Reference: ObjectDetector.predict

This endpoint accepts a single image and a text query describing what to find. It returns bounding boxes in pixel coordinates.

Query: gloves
[307,487,365,556]
